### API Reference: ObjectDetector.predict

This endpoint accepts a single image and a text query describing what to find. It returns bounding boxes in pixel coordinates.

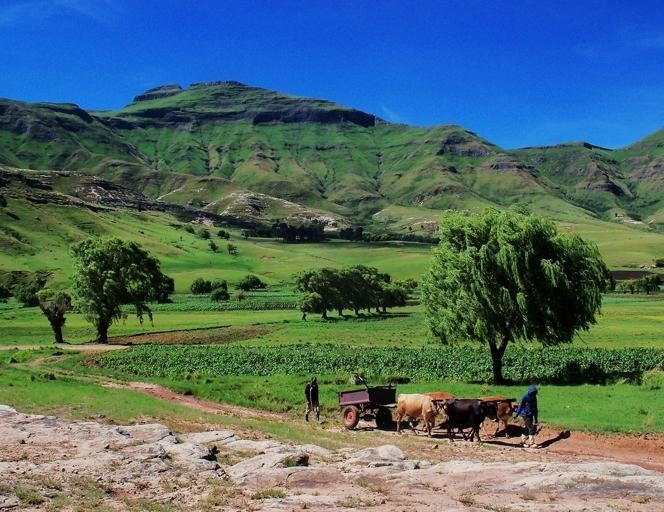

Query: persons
[305,376,320,421]
[513,386,538,448]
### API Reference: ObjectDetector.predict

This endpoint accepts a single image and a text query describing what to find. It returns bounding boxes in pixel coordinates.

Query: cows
[396,392,443,439]
[420,392,455,434]
[445,397,498,444]
[477,395,516,439]
